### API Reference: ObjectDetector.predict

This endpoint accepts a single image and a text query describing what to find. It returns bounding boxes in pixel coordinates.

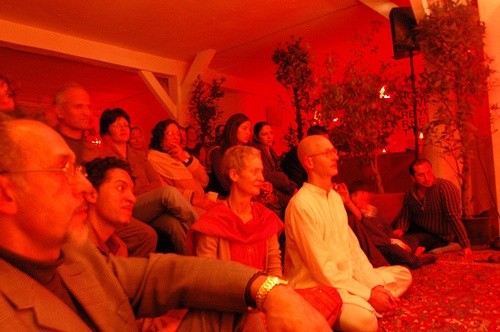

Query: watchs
[256,275,288,313]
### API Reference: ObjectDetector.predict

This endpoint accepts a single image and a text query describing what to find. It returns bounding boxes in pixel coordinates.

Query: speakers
[390,7,422,60]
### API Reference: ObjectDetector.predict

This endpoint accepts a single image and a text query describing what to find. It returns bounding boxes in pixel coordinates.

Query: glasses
[0,161,87,184]
[164,130,181,136]
[308,148,338,158]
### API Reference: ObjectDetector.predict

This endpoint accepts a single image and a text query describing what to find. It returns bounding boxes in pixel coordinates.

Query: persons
[331,182,437,269]
[0,118,336,332]
[145,118,213,210]
[179,123,227,175]
[51,85,197,254]
[254,120,298,217]
[97,105,158,197]
[0,75,17,113]
[128,127,144,150]
[208,113,254,199]
[283,135,412,331]
[185,146,341,332]
[80,157,158,259]
[390,159,470,257]
